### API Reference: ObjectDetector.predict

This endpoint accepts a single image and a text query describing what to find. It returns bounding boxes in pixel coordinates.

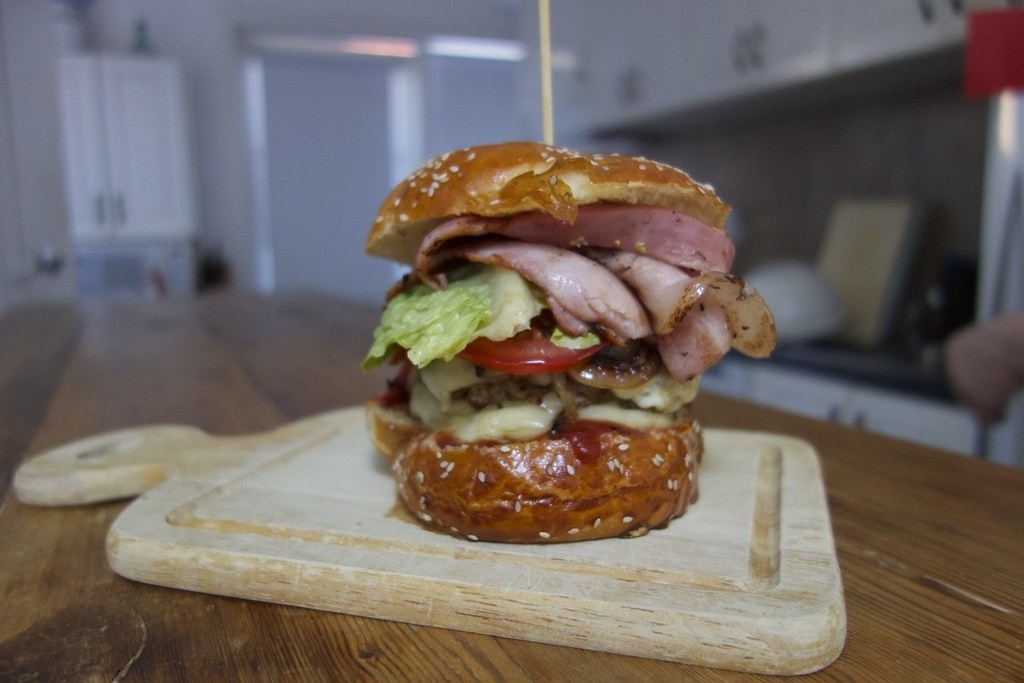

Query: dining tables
[0,283,1024,682]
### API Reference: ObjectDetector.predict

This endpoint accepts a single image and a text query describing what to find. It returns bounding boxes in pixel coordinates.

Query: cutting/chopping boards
[14,402,847,676]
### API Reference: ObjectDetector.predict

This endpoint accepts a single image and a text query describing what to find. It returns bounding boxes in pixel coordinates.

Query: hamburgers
[363,142,777,547]
